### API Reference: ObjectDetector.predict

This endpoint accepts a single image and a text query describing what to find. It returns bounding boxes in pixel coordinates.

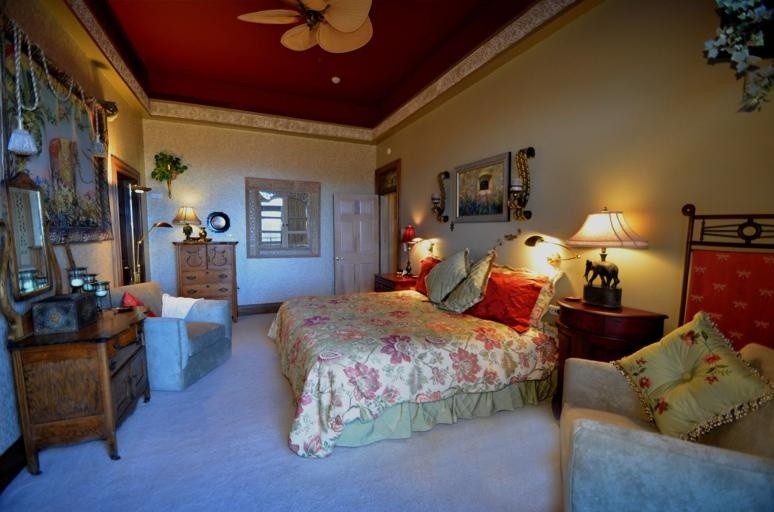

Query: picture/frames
[450,149,516,224]
[0,8,120,246]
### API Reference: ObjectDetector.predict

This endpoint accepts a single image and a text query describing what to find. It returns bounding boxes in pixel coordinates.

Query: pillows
[421,247,471,306]
[442,250,496,314]
[610,307,773,440]
[120,291,156,318]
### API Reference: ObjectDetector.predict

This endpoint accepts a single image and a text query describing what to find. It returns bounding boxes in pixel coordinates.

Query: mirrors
[1,169,54,303]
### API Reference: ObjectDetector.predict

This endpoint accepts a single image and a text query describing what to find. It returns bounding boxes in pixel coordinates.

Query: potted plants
[150,151,188,199]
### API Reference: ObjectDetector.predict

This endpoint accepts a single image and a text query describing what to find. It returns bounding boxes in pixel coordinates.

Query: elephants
[584,260,620,289]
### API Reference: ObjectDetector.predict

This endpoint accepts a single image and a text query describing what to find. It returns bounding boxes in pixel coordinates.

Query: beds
[267,288,557,461]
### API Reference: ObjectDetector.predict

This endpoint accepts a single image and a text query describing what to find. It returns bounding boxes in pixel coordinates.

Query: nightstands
[373,272,419,293]
[552,297,668,422]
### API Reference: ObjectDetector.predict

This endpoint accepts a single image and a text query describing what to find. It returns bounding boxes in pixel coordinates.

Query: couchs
[98,280,232,393]
[556,341,773,512]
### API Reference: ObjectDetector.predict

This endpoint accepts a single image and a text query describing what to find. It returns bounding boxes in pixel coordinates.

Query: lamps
[428,170,451,224]
[507,145,535,221]
[565,205,649,311]
[234,0,377,54]
[522,233,583,270]
[397,223,417,278]
[128,183,172,285]
[172,204,201,242]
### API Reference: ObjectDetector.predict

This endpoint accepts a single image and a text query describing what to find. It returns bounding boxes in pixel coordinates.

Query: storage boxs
[30,292,99,337]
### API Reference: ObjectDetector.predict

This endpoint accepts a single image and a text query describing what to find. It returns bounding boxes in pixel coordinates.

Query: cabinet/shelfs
[174,239,241,329]
[8,304,155,474]
